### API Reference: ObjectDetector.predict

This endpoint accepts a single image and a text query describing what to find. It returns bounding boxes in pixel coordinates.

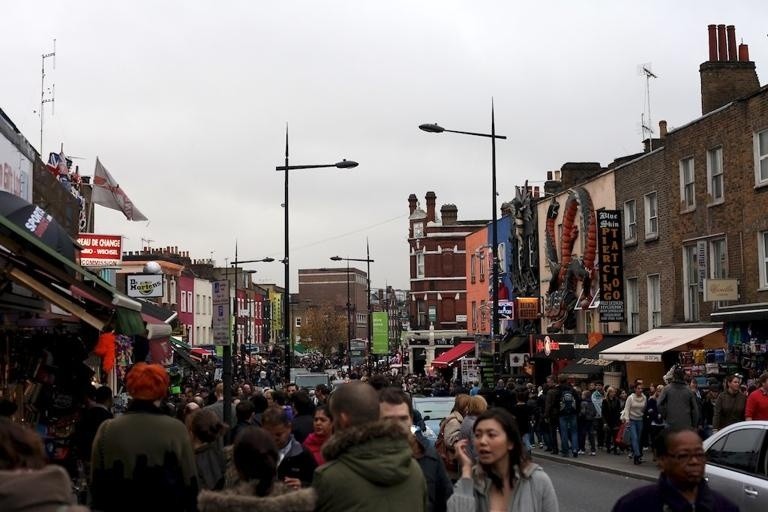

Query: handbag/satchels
[434,436,454,472]
[614,422,632,447]
[620,409,626,421]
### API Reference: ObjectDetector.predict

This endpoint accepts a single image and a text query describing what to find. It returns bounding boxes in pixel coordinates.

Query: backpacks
[559,390,576,415]
[584,399,597,421]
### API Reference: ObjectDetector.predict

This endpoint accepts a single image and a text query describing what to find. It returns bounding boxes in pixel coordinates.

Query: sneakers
[529,440,648,466]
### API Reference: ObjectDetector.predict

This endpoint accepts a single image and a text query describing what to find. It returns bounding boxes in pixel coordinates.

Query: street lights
[329,237,373,379]
[221,257,256,372]
[231,240,275,383]
[274,120,362,385]
[242,290,276,373]
[419,94,510,381]
[318,255,355,375]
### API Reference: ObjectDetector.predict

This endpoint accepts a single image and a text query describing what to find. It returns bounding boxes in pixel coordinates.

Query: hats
[124,361,169,401]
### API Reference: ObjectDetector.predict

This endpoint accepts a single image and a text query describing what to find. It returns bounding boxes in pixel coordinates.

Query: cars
[286,367,351,408]
[387,364,409,377]
[411,396,457,449]
[701,420,767,512]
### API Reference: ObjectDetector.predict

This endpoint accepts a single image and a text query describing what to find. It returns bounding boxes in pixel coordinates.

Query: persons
[1,346,768,512]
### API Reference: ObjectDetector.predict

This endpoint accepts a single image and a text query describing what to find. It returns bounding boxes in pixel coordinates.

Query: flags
[91,159,148,222]
[45,153,72,183]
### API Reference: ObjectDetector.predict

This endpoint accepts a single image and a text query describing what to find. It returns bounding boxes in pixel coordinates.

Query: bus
[346,338,368,368]
